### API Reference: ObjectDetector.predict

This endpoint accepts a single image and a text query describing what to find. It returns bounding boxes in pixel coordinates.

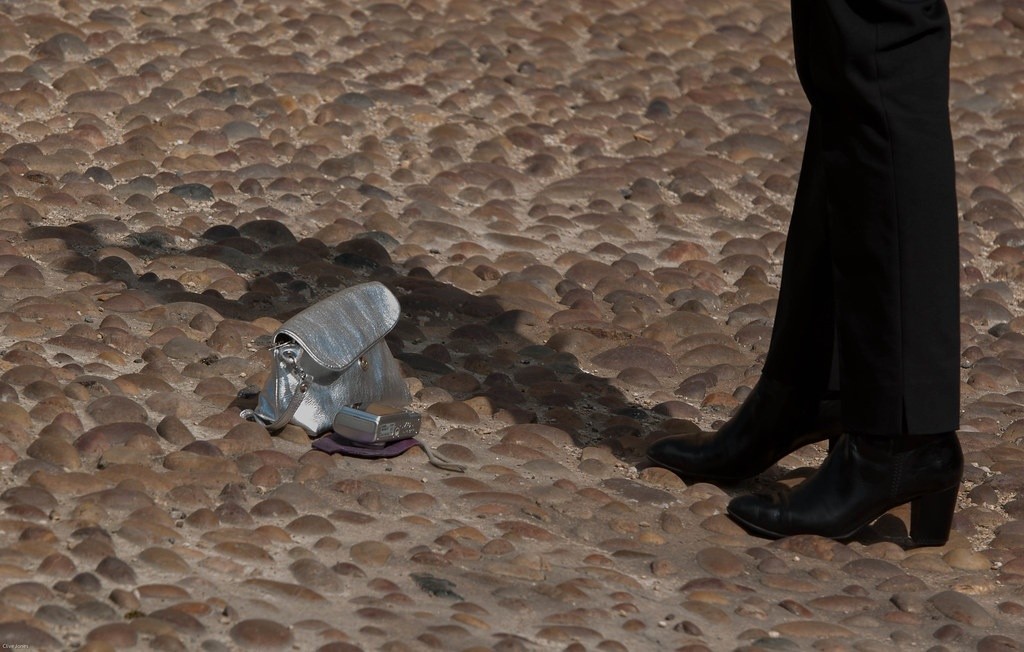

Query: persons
[645,0,964,546]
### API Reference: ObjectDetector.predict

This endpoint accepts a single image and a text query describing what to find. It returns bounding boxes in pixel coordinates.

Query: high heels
[727,431,964,546]
[644,372,852,482]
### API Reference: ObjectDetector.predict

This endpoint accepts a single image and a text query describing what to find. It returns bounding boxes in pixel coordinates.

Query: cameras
[331,402,421,451]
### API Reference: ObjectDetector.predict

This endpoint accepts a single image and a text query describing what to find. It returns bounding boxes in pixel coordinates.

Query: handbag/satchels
[239,281,411,436]
